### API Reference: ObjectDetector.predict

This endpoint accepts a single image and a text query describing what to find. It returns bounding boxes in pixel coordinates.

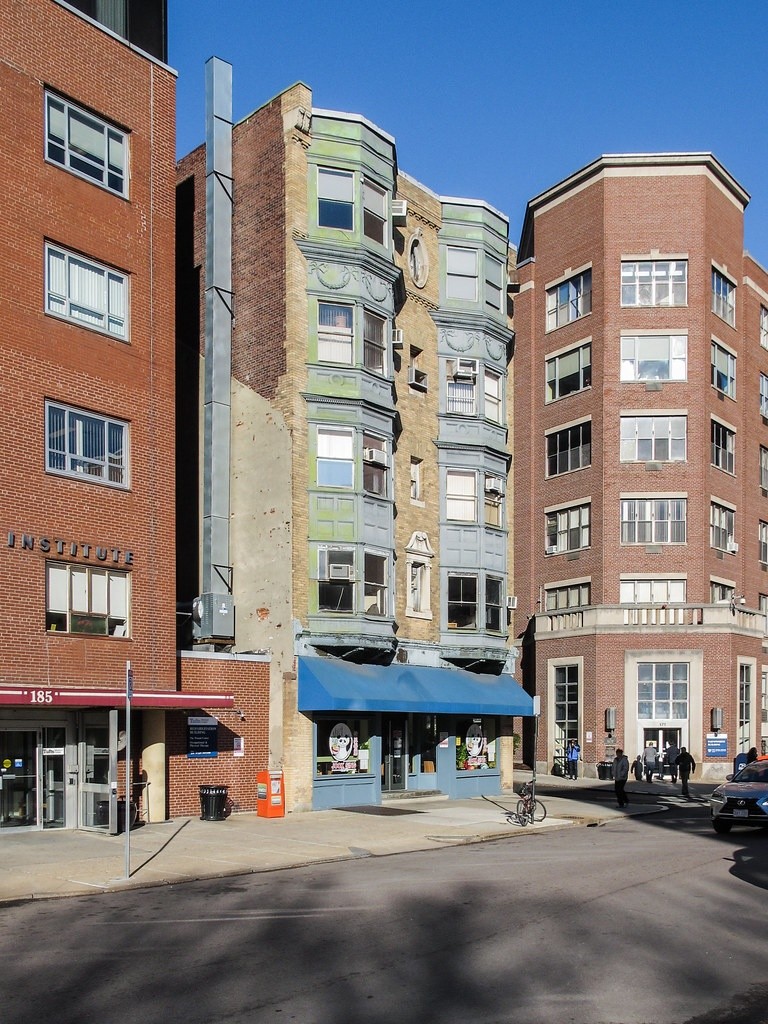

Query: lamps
[731,594,746,605]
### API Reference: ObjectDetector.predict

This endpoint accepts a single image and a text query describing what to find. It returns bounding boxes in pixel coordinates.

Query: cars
[710,759,768,833]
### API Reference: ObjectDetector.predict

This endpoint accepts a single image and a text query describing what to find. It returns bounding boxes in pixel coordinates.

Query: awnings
[298,655,536,716]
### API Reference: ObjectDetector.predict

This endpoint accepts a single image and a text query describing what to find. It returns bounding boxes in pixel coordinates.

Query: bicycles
[516,779,547,826]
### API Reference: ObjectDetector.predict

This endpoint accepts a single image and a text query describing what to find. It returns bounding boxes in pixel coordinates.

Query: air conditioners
[486,478,505,497]
[392,200,408,227]
[507,596,517,609]
[368,449,386,465]
[392,329,403,349]
[727,542,738,552]
[329,564,350,580]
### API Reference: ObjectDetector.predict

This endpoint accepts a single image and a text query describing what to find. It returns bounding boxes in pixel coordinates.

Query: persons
[642,742,657,783]
[675,747,696,795]
[566,740,580,780]
[746,747,768,777]
[666,741,681,782]
[612,749,630,807]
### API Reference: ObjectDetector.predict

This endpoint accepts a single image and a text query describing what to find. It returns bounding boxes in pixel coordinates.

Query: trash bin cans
[197,785,230,821]
[599,761,615,781]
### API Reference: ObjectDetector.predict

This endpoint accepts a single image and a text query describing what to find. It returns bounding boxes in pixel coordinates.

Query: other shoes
[647,779,653,783]
[670,777,677,783]
